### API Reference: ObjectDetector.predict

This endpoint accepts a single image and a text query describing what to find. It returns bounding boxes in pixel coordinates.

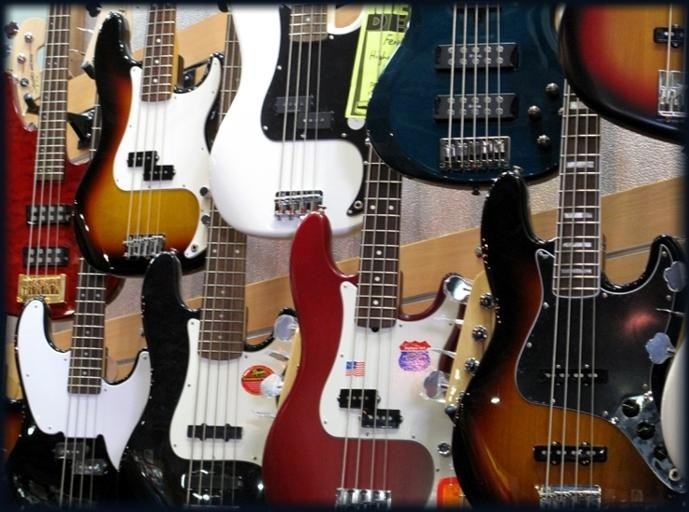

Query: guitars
[258,2,477,512]
[365,0,574,193]
[2,7,153,512]
[107,14,300,511]
[206,1,378,241]
[449,74,688,511]
[1,0,126,321]
[69,3,228,281]
[557,1,688,147]
[445,268,499,422]
